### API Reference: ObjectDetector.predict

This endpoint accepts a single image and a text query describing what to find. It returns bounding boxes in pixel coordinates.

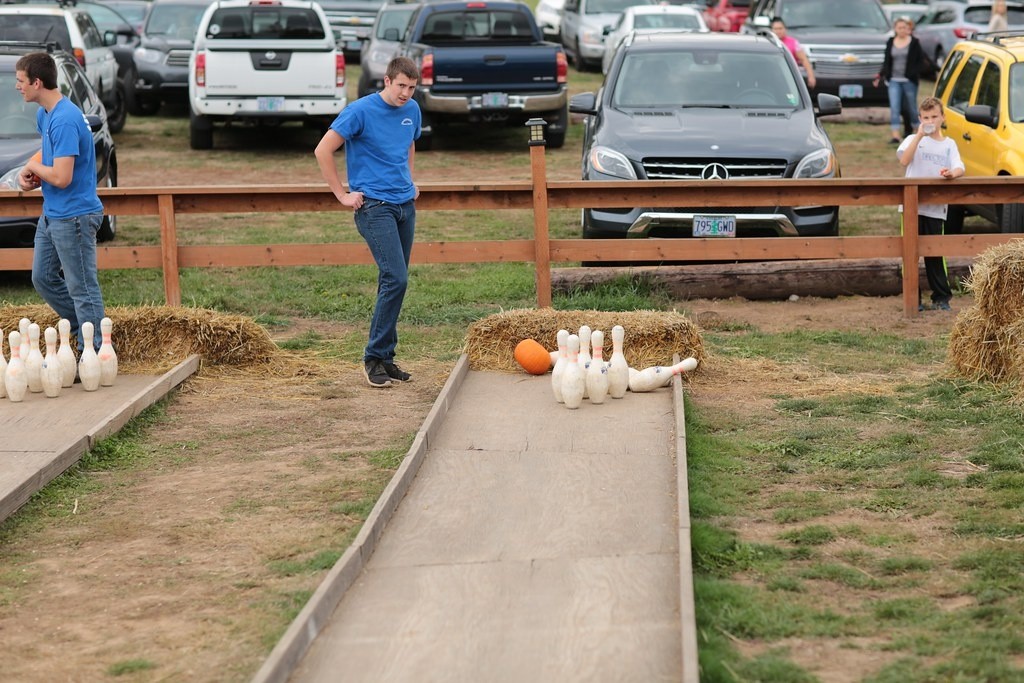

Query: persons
[313,56,423,387]
[873,16,941,145]
[15,52,105,384]
[989,0,1009,37]
[769,17,816,90]
[895,97,965,312]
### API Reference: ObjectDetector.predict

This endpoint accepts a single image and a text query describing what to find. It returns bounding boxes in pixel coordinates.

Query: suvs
[315,0,395,63]
[558,0,660,72]
[356,4,420,102]
[570,29,842,267]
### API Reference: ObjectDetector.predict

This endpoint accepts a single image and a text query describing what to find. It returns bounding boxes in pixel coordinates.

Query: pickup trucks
[188,1,347,150]
[384,1,569,152]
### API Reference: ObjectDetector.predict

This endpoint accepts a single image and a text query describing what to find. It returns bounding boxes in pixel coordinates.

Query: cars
[0,40,118,287]
[932,30,1024,233]
[883,0,1024,82]
[535,0,563,43]
[681,0,753,34]
[599,4,724,76]
[739,0,897,109]
[0,0,217,116]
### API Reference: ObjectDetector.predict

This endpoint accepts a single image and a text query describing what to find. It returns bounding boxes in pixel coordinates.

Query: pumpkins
[514,339,552,375]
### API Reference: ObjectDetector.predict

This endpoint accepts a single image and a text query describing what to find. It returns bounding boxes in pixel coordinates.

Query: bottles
[923,123,937,134]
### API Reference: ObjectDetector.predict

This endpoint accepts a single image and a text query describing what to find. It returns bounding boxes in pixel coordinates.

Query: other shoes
[888,137,899,144]
[932,298,950,311]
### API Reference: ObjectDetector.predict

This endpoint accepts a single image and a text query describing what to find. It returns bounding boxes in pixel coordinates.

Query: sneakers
[384,358,412,382]
[364,354,392,387]
